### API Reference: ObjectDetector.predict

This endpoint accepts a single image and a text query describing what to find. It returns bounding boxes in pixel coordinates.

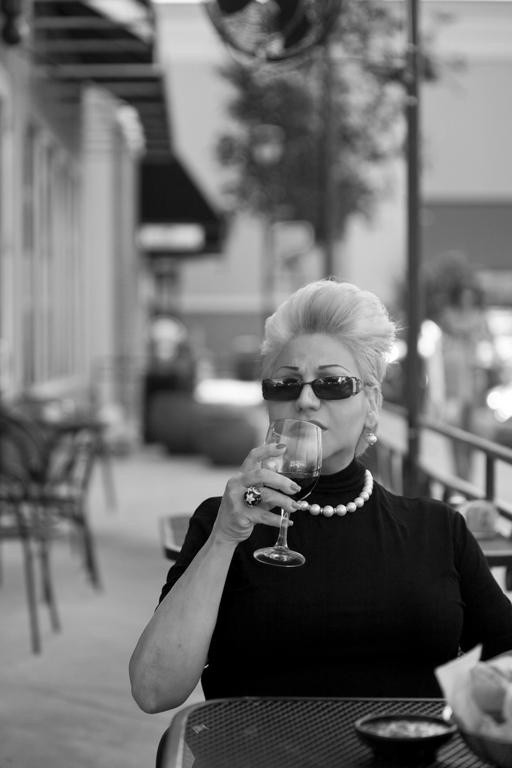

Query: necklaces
[281,468,375,517]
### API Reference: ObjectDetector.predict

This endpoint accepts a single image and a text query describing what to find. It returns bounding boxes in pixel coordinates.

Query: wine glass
[252,416,324,569]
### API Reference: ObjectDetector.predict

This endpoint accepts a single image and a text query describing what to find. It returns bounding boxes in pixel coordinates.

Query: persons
[130,279,512,767]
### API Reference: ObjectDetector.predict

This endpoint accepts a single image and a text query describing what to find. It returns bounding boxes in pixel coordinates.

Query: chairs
[28,415,104,628]
[2,409,59,651]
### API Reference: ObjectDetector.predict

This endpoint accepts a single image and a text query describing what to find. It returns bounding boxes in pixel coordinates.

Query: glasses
[263,373,367,401]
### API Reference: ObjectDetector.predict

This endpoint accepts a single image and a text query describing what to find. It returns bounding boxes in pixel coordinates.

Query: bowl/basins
[352,710,457,764]
[438,698,510,764]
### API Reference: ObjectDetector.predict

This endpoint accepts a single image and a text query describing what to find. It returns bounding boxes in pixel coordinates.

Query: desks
[162,506,512,575]
[157,694,511,767]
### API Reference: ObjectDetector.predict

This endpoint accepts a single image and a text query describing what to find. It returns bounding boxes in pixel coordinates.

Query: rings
[245,485,262,506]
